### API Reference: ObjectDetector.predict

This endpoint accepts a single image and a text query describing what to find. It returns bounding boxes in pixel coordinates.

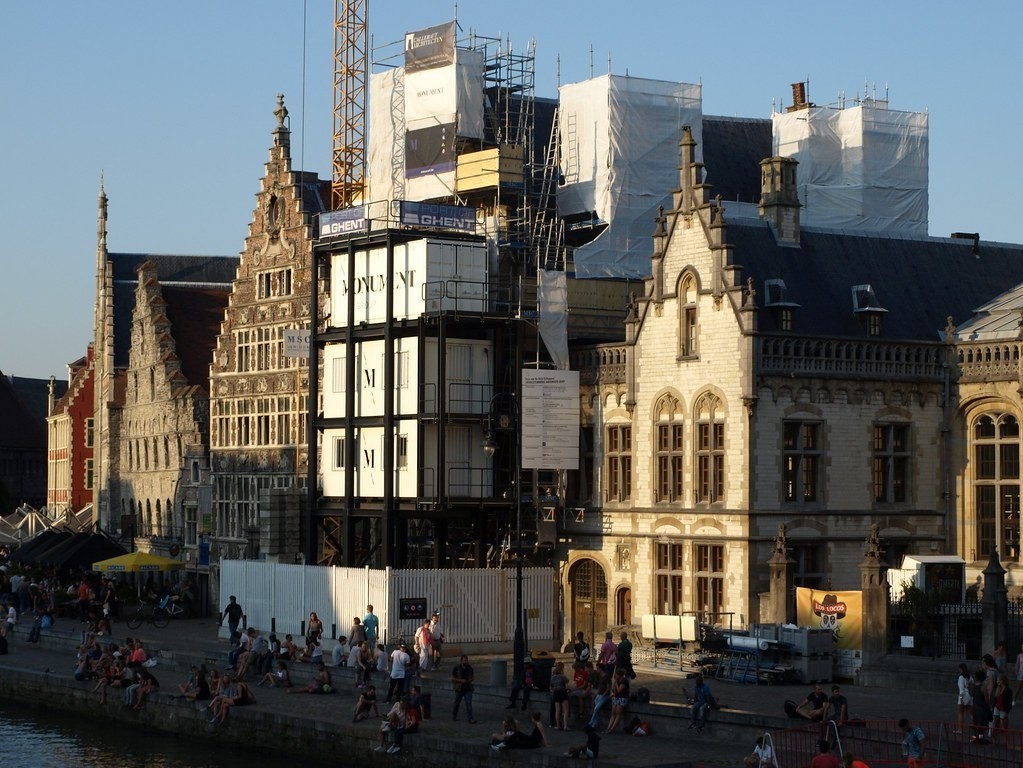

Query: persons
[796,685,850,748]
[896,719,927,768]
[0,549,119,650]
[688,676,717,734]
[222,596,478,752]
[743,736,869,768]
[74,636,159,710]
[178,665,255,725]
[953,641,1023,743]
[490,629,638,760]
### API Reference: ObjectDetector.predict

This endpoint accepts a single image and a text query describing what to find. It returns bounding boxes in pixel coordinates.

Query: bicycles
[126,598,170,631]
[152,592,191,620]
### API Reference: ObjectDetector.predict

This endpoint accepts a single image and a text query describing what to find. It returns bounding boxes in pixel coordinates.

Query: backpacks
[576,642,590,661]
[604,643,616,665]
[784,700,802,719]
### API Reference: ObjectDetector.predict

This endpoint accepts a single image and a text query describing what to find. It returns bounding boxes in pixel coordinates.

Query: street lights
[480,390,524,687]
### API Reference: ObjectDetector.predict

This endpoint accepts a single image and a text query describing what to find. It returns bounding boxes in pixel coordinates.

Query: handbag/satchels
[625,717,650,737]
[452,665,461,690]
[983,706,994,722]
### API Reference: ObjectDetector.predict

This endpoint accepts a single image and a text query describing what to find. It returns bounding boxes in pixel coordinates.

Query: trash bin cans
[532,652,556,690]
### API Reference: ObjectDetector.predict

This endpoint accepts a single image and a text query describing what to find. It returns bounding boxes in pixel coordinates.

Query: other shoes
[210,717,218,723]
[25,639,32,642]
[201,707,207,713]
[696,727,702,734]
[373,745,385,752]
[469,718,477,724]
[505,702,515,709]
[30,639,39,643]
[387,743,400,754]
[521,705,527,710]
[688,722,696,729]
[549,711,615,735]
[354,681,365,688]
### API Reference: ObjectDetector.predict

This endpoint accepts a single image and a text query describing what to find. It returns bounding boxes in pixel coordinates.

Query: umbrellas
[94,551,182,596]
[19,531,121,560]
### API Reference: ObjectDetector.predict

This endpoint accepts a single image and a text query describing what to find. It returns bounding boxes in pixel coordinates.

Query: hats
[524,656,532,663]
